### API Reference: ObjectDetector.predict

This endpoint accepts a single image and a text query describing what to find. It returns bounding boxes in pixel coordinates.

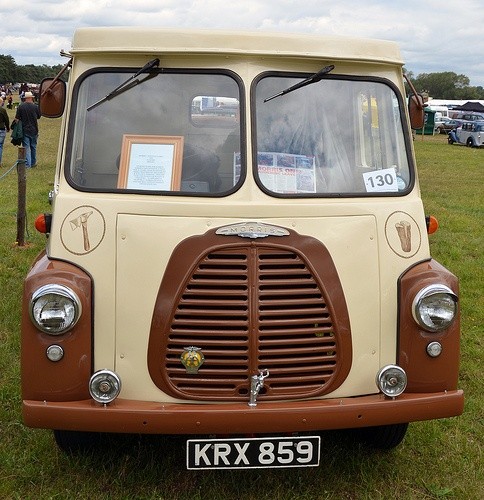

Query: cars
[433,112,484,148]
[21,25,465,471]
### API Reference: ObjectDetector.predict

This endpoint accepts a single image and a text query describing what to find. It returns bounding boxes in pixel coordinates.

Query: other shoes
[31,164,36,167]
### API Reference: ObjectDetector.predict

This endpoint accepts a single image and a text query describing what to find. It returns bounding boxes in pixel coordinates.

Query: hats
[23,92,35,98]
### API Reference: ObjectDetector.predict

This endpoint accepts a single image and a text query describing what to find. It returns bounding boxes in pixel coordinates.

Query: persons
[14,92,41,167]
[0,83,40,109]
[0,98,10,167]
